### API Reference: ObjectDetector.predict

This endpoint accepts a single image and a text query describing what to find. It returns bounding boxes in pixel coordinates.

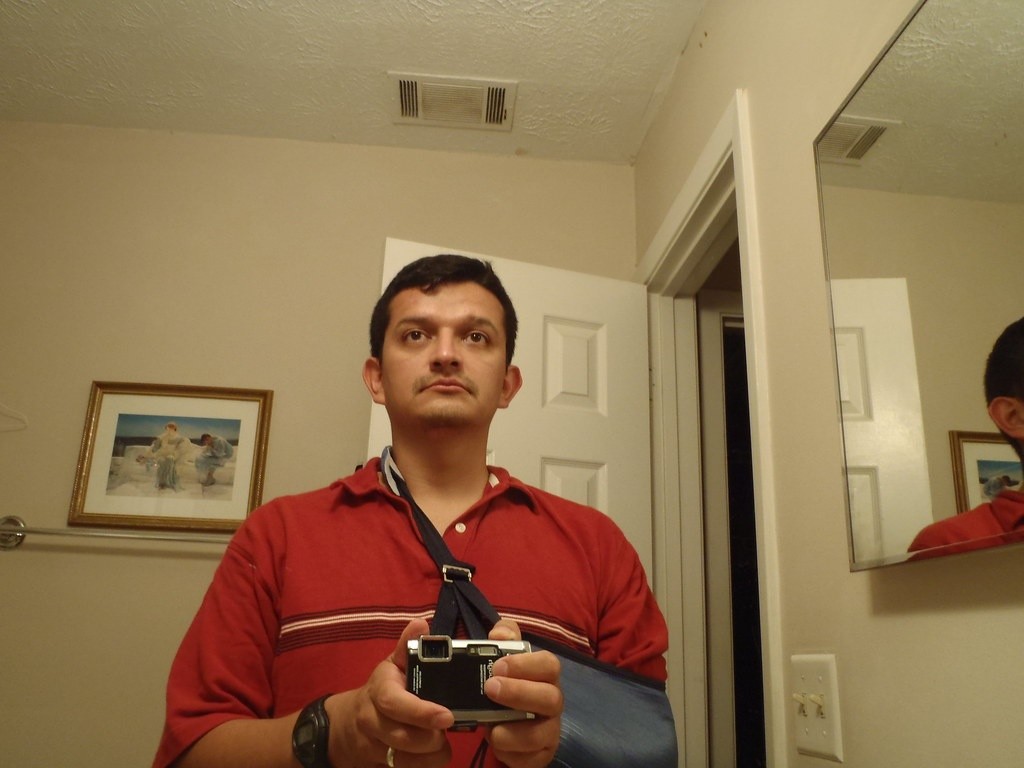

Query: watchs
[291,693,335,768]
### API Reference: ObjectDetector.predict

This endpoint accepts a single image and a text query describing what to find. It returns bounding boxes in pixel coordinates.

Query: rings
[386,746,395,767]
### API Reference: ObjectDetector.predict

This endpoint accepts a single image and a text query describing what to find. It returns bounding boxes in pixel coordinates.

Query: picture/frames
[65,381,273,531]
[948,427,1023,513]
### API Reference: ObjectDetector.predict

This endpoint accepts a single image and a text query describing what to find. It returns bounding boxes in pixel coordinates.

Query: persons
[907,317,1024,562]
[152,422,184,491]
[150,255,680,768]
[195,433,232,486]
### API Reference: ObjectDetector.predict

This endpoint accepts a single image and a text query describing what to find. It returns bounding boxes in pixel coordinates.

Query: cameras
[406,633,536,729]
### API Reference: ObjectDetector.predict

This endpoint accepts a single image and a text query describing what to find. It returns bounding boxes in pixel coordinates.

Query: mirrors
[812,0,1024,572]
